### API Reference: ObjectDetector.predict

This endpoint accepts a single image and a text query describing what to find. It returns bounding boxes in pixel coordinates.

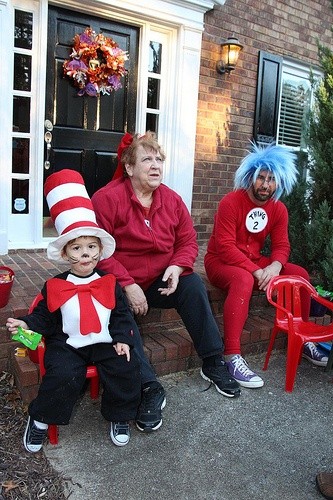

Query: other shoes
[316,472,332,499]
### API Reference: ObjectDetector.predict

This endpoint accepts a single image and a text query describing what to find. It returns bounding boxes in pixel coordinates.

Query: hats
[46,169,116,262]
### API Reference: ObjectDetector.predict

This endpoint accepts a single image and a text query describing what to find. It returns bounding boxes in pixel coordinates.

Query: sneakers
[110,419,131,446]
[199,360,243,398]
[136,382,167,433]
[300,341,329,366]
[225,354,264,388]
[23,416,49,453]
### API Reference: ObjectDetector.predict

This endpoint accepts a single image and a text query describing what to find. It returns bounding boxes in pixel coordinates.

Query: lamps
[216,32,244,79]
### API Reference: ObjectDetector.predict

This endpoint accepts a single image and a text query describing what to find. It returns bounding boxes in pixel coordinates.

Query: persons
[204,136,329,388]
[89,130,242,433]
[5,170,141,453]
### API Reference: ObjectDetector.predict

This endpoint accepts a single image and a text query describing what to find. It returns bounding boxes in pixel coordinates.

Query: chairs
[28,293,99,445]
[262,275,333,393]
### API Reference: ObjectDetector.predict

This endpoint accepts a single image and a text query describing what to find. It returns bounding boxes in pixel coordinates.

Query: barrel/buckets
[0,265,15,308]
